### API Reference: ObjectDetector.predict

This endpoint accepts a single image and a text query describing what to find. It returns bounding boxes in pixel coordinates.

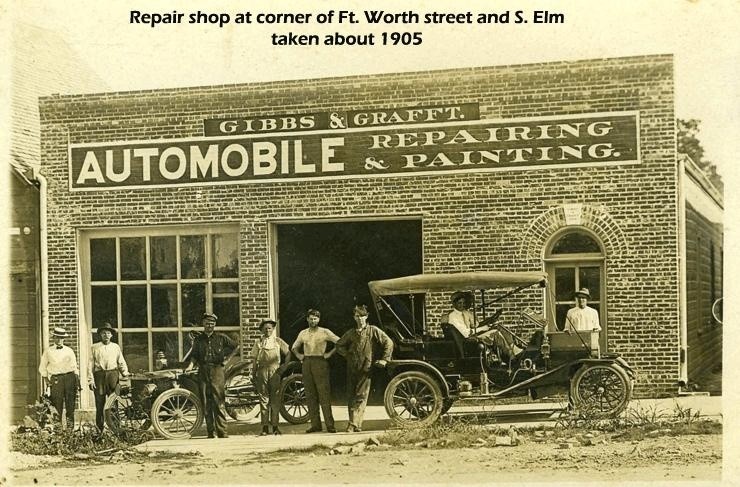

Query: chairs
[441,320,487,358]
[387,324,427,358]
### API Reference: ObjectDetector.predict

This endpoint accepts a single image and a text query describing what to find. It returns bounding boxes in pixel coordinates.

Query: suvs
[365,270,633,427]
[104,338,324,442]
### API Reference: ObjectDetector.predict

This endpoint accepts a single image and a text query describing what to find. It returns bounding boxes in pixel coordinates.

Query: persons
[87,322,130,432]
[193,313,237,438]
[291,309,341,433]
[336,304,394,432]
[251,318,291,436]
[448,292,523,364]
[38,328,81,429]
[563,287,599,355]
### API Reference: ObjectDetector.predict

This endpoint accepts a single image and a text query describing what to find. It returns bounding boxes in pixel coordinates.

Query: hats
[576,288,591,298]
[451,292,465,303]
[97,323,116,334]
[51,328,68,336]
[259,319,276,331]
